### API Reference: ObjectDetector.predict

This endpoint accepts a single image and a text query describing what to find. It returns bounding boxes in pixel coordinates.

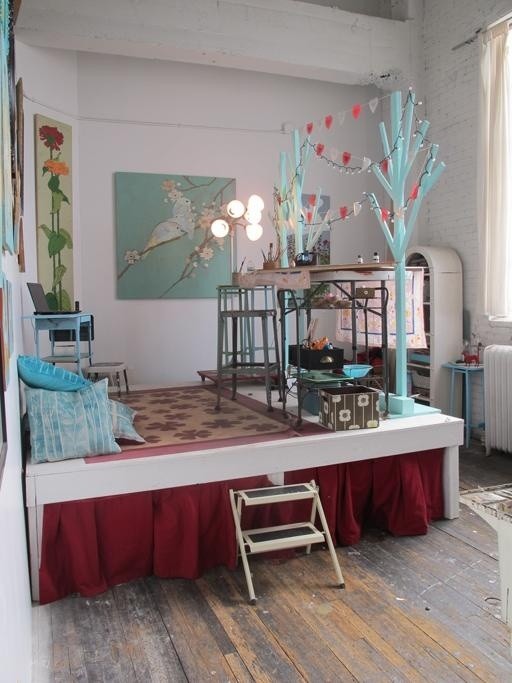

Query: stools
[213,309,285,415]
[217,281,280,373]
[87,361,130,398]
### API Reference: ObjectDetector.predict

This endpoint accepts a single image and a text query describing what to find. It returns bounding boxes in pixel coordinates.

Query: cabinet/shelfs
[227,477,346,603]
[398,244,464,418]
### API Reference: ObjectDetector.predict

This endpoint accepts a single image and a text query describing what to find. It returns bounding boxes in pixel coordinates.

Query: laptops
[27,282,80,314]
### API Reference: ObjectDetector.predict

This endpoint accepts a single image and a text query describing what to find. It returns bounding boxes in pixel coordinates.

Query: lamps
[209,192,267,269]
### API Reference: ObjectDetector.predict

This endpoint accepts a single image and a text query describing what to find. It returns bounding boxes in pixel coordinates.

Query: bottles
[75,301,79,311]
[357,254,363,264]
[372,251,379,263]
[329,343,333,349]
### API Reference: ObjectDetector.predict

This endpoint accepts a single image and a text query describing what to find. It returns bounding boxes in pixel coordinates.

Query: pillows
[110,398,146,445]
[25,375,123,466]
[17,354,95,391]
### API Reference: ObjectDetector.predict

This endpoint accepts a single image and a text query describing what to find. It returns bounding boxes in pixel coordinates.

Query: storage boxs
[289,343,345,369]
[317,385,380,431]
[291,372,353,416]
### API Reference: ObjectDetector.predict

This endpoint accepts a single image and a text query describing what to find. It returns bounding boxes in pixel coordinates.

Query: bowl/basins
[343,364,375,379]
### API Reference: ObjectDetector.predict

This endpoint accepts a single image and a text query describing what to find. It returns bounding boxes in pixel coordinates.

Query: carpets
[83,380,339,465]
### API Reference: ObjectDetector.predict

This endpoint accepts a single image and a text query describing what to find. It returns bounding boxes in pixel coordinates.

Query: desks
[460,479,512,628]
[239,262,427,428]
[441,362,484,448]
[31,311,95,379]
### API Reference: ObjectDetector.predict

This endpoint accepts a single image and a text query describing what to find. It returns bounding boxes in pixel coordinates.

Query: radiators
[483,344,511,456]
